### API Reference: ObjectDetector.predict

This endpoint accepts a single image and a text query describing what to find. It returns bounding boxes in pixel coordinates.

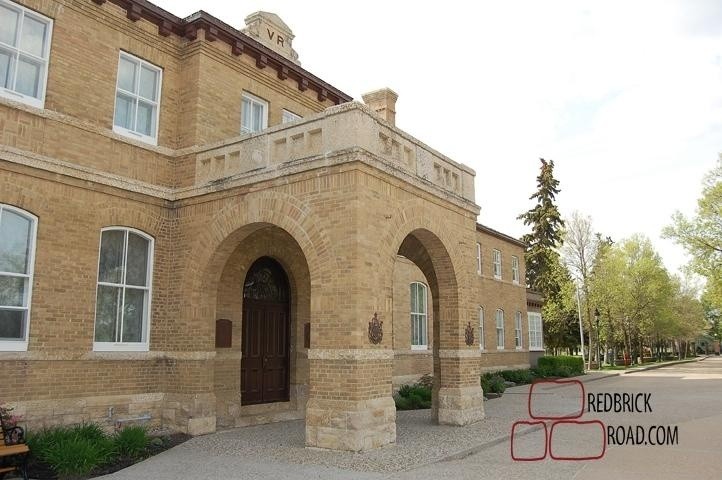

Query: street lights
[594,308,603,370]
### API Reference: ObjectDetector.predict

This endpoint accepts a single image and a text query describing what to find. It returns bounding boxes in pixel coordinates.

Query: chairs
[0,422,31,479]
